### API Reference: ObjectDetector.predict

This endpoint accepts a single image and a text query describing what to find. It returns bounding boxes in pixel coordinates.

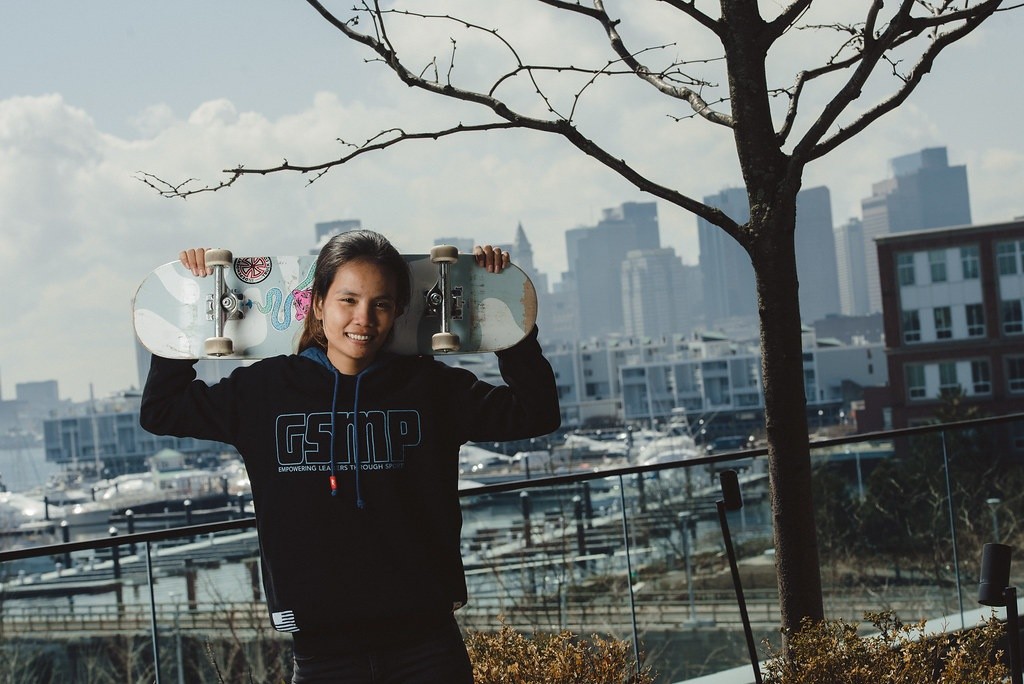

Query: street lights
[679,509,696,627]
[987,497,1002,545]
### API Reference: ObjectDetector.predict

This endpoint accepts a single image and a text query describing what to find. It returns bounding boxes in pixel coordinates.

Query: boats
[2,380,253,537]
[456,405,715,513]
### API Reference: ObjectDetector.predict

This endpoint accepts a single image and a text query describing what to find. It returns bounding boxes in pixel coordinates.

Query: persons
[139,229,561,684]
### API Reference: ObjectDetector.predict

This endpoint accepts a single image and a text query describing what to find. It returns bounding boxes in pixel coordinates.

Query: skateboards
[133,242,539,360]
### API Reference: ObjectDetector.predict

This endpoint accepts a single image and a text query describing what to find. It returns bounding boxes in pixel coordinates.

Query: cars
[703,432,759,474]
